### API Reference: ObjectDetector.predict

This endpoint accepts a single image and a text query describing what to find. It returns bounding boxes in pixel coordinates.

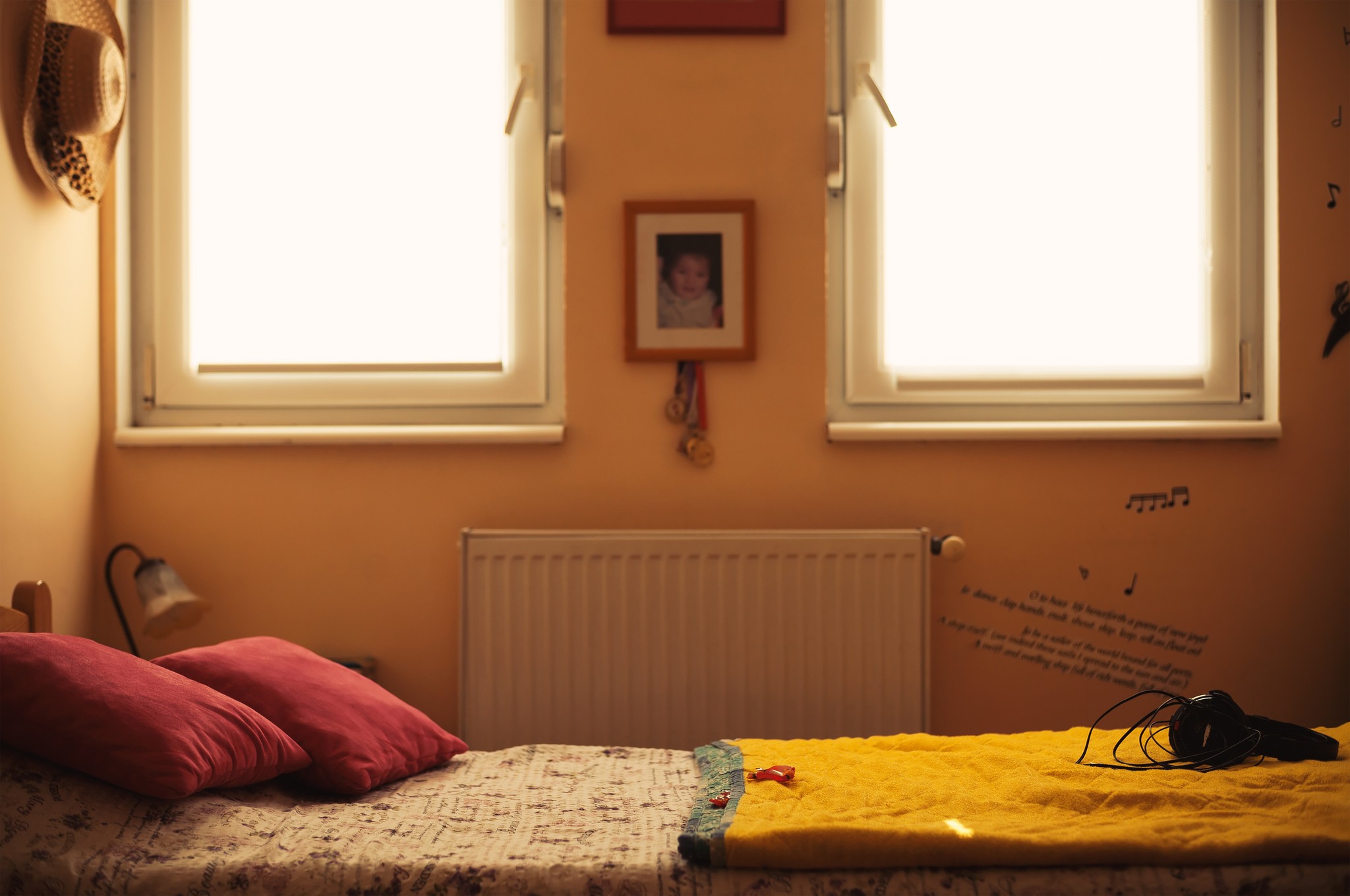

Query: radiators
[450,519,967,763]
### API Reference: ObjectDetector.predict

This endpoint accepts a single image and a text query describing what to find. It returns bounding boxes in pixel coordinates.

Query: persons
[654,240,725,330]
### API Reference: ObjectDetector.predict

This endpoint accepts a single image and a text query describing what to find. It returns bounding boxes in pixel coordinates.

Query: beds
[0,573,1350,895]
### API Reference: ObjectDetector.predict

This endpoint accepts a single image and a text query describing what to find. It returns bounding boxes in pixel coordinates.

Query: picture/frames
[605,1,788,38]
[620,192,761,366]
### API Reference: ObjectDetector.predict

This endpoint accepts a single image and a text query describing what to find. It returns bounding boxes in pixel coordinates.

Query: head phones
[1168,690,1339,768]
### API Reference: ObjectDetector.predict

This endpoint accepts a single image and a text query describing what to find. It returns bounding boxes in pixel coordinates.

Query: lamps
[105,542,205,661]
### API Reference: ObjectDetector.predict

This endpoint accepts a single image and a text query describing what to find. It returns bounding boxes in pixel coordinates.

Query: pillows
[0,628,314,809]
[144,634,471,802]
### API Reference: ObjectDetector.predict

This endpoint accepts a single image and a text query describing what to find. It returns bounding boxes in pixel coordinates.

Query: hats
[24,0,129,211]
